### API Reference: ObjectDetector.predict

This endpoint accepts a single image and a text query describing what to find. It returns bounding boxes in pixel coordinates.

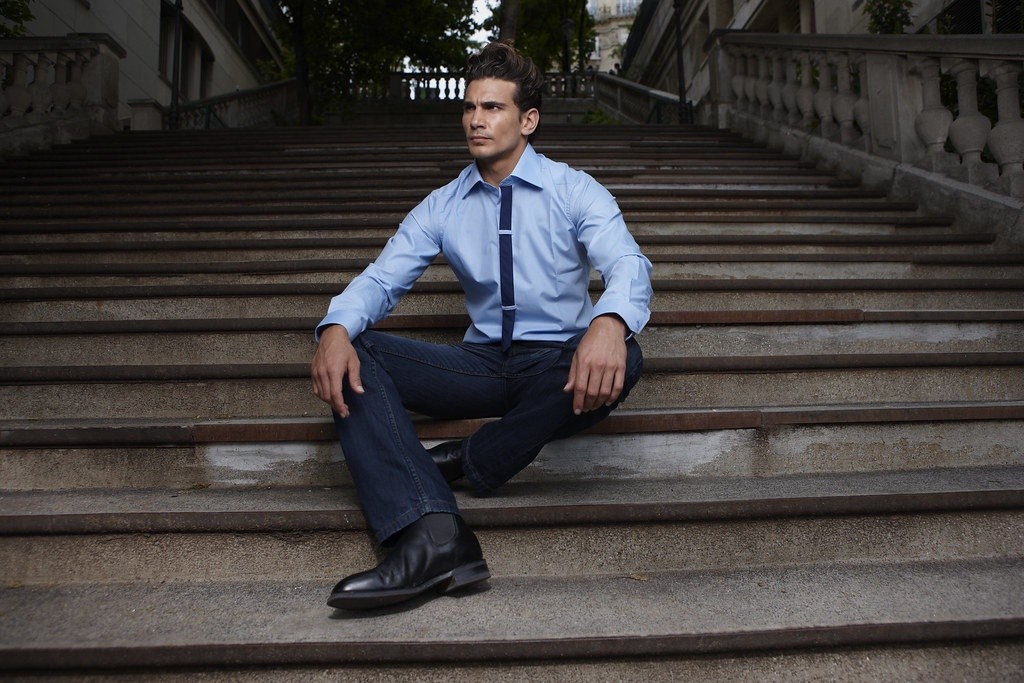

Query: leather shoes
[425,440,466,483]
[326,512,491,609]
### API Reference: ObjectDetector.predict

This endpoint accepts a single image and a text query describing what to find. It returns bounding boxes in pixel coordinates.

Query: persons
[310,43,655,611]
